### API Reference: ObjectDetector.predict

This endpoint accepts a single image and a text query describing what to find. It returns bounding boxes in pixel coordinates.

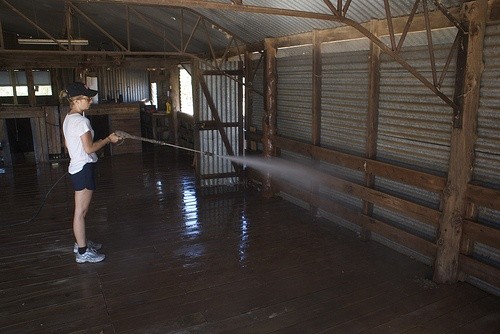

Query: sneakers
[75,246,105,263]
[73,238,102,253]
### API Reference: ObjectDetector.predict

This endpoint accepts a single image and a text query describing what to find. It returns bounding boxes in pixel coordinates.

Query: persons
[57,80,125,264]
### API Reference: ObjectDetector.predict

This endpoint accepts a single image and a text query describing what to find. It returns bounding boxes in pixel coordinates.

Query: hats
[66,82,98,97]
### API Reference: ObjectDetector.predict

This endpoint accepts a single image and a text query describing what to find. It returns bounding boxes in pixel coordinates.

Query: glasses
[82,97,90,102]
